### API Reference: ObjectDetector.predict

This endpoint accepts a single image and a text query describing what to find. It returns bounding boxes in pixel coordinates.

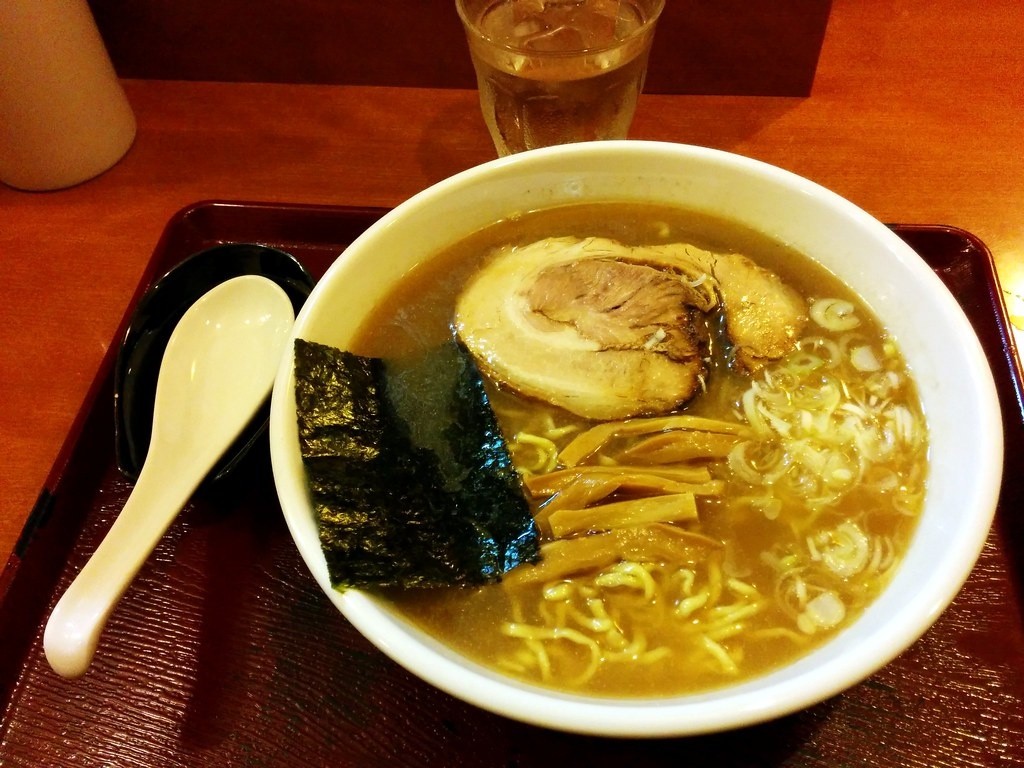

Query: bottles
[0,0,137,197]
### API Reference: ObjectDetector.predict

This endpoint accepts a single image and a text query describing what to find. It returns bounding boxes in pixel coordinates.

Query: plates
[114,239,317,494]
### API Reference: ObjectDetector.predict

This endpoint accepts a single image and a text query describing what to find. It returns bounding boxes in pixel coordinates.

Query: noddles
[345,202,924,701]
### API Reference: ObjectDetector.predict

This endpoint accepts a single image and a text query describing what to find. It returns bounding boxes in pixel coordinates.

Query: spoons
[40,270,293,677]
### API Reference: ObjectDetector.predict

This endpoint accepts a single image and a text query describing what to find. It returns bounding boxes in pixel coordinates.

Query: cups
[456,2,667,160]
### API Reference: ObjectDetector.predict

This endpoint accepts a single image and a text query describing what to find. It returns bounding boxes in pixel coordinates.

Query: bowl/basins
[267,136,1008,746]
